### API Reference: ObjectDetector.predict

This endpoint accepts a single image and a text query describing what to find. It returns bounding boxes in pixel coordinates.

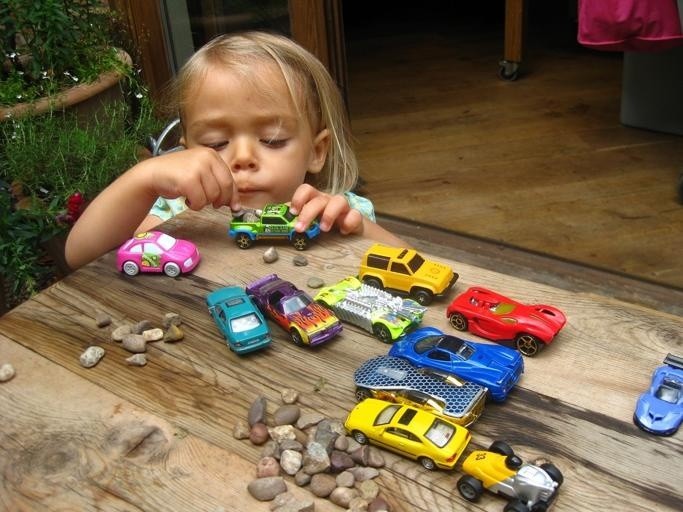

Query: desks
[0,198,683,512]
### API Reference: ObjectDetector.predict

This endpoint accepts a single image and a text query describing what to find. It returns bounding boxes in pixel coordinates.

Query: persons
[64,30,412,267]
